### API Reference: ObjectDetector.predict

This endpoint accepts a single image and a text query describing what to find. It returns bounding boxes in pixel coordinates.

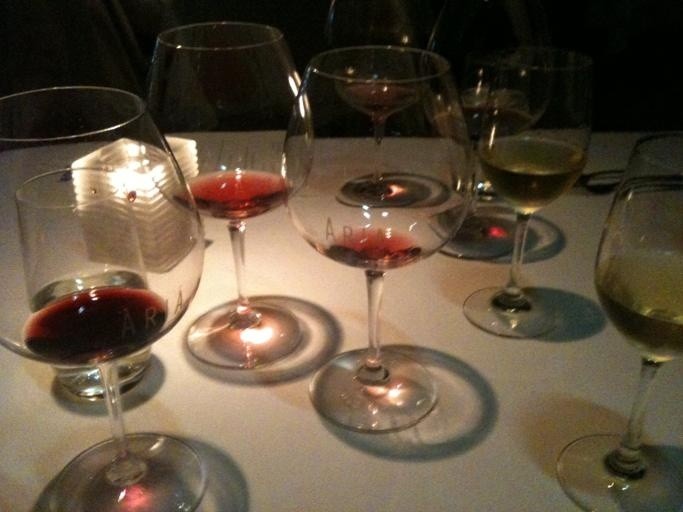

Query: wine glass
[421,0,600,341]
[326,0,449,209]
[142,23,344,390]
[278,43,474,435]
[0,83,211,512]
[553,131,683,512]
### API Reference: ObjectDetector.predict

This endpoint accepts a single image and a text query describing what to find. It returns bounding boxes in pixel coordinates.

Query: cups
[12,166,152,402]
